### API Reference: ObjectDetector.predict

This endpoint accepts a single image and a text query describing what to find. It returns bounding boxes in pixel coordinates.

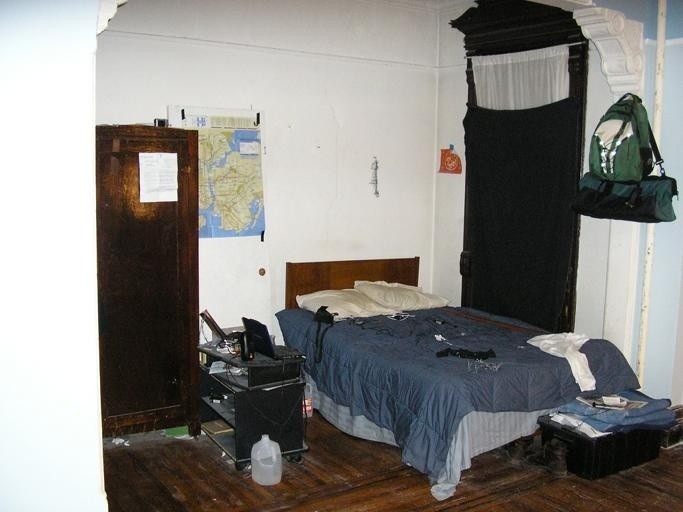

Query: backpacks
[588,92,656,185]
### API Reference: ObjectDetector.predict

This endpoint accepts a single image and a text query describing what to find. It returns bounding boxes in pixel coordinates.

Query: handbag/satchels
[567,95,678,224]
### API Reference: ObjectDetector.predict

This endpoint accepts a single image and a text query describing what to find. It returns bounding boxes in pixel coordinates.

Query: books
[575,393,648,412]
[200,418,235,435]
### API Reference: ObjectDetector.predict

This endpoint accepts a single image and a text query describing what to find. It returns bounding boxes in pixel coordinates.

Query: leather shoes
[504,440,523,465]
[525,438,568,479]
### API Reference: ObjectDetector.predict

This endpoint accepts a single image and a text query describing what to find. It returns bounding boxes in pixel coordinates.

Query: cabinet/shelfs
[196,342,308,472]
[94,124,200,441]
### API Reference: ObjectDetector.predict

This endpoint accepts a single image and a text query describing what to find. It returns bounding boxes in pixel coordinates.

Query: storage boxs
[535,387,666,480]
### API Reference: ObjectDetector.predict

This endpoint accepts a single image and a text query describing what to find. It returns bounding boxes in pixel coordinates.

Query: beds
[273,256,643,500]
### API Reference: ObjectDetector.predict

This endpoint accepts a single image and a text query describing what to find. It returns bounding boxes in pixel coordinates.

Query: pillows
[354,278,450,311]
[295,288,400,322]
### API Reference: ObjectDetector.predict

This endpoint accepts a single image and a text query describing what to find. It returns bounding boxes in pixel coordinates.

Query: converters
[212,395,224,404]
[433,319,444,324]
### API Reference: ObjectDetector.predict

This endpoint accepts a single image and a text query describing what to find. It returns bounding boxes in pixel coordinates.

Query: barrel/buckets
[250,434,282,486]
[302,383,314,419]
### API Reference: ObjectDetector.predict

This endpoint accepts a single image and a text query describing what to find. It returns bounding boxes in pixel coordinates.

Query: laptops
[241,317,306,361]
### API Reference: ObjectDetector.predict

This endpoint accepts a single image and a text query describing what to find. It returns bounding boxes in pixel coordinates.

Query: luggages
[536,414,662,481]
